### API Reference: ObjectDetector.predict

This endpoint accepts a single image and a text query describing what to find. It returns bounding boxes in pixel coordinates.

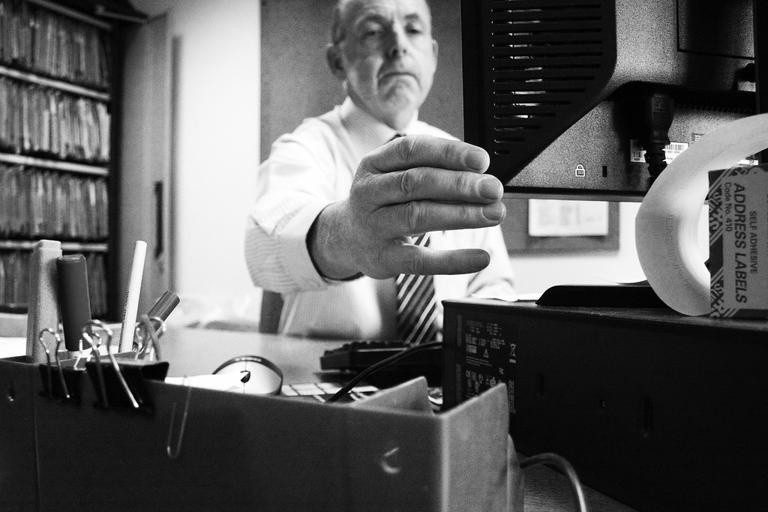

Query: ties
[384,134,441,349]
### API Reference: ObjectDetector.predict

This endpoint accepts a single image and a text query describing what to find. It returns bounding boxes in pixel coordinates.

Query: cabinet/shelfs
[3,1,120,320]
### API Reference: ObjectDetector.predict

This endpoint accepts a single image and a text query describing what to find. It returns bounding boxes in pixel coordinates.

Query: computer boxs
[440,303,766,512]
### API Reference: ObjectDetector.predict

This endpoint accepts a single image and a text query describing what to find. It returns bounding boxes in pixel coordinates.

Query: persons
[246,0,514,350]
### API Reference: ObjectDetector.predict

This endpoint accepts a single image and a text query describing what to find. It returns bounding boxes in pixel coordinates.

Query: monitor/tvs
[461,0,768,305]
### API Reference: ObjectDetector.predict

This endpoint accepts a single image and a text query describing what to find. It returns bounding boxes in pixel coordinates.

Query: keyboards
[320,337,442,389]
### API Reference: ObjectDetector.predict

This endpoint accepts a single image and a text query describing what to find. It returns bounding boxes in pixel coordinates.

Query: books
[0,0,115,319]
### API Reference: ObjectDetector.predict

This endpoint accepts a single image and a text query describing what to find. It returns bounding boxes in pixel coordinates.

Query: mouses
[211,354,284,397]
